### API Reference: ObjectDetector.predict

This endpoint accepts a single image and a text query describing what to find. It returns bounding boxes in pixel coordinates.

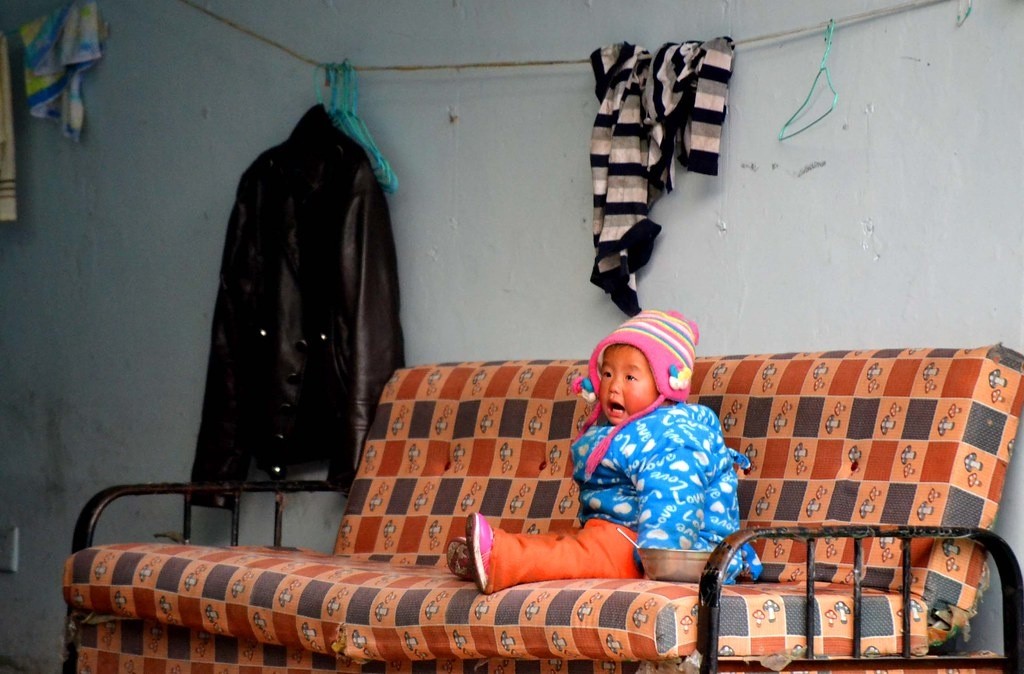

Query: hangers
[774,15,838,143]
[305,55,400,203]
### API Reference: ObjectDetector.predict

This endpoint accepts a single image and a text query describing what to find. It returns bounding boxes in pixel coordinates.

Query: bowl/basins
[635,545,715,583]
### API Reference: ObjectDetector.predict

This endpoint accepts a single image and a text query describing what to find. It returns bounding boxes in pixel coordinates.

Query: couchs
[56,343,1023,674]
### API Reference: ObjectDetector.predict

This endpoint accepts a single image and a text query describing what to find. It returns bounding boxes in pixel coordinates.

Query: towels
[0,0,116,222]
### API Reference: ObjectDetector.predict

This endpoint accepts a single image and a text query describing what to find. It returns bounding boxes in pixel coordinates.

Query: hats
[570,309,700,484]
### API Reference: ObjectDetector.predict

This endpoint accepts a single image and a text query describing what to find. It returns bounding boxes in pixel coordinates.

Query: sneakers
[445,535,475,582]
[465,512,494,593]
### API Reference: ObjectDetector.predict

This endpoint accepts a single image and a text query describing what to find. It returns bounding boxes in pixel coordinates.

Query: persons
[447,310,761,593]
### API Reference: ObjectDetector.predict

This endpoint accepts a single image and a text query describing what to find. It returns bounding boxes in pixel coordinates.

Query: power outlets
[0,524,19,574]
